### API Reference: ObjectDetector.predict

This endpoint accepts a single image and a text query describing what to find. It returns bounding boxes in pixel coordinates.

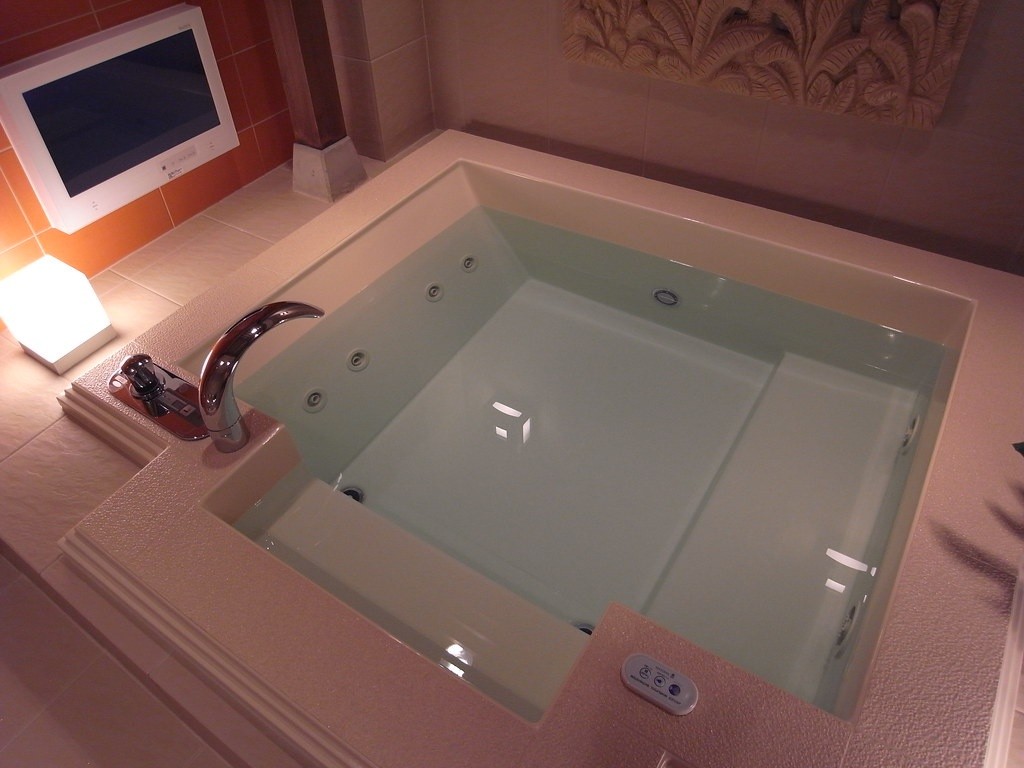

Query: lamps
[0,253,116,377]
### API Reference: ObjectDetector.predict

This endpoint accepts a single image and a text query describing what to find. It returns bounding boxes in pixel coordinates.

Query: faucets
[197,299,326,455]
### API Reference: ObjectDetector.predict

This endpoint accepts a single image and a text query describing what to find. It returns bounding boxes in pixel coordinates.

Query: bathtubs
[54,124,1022,768]
